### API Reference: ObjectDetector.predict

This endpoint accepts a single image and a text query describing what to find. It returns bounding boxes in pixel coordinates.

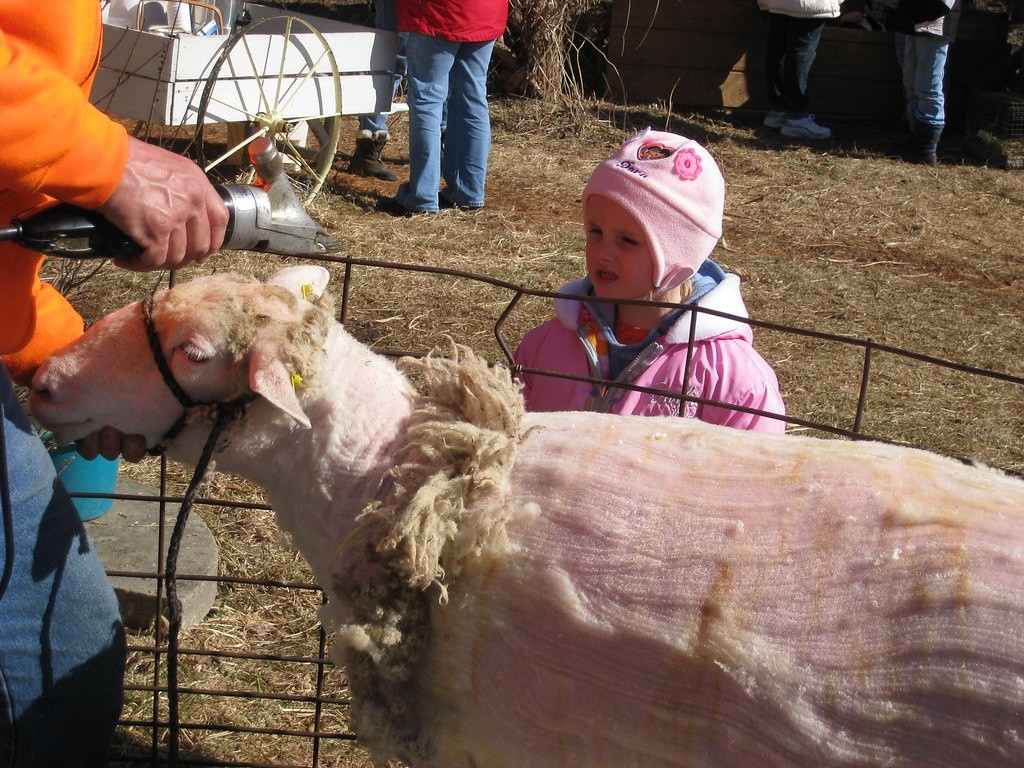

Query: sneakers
[780,114,831,140]
[764,110,783,128]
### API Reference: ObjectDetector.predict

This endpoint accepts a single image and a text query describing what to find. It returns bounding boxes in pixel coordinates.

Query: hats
[581,129,725,302]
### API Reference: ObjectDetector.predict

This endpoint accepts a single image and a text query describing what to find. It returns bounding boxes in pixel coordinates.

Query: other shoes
[377,194,432,218]
[437,192,481,210]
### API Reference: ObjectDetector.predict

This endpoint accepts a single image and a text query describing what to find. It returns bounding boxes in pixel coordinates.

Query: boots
[348,130,396,180]
[887,119,943,165]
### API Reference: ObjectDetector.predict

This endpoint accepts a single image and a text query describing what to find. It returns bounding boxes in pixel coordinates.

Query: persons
[757,0,845,140]
[825,0,899,31]
[349,0,509,217]
[0,0,230,768]
[513,127,785,435]
[884,0,961,166]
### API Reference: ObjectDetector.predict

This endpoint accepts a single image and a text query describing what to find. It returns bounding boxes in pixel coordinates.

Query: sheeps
[29,264,1023,768]
